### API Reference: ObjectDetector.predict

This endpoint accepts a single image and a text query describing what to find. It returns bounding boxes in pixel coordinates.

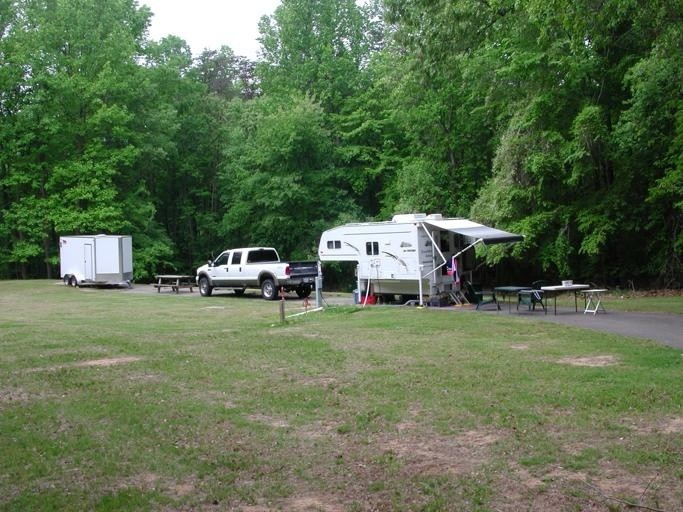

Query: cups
[562,280,573,286]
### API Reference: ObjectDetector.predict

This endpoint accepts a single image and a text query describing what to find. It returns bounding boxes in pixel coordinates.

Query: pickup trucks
[196,247,318,300]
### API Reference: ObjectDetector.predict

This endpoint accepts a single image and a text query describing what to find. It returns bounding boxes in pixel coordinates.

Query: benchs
[173,282,197,292]
[154,285,179,293]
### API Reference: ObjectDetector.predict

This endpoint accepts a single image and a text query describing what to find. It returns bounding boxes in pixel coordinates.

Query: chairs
[465,280,501,310]
[517,280,547,312]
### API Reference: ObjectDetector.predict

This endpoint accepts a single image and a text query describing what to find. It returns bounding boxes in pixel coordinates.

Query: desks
[494,286,531,313]
[583,289,608,315]
[541,284,590,315]
[154,275,193,293]
